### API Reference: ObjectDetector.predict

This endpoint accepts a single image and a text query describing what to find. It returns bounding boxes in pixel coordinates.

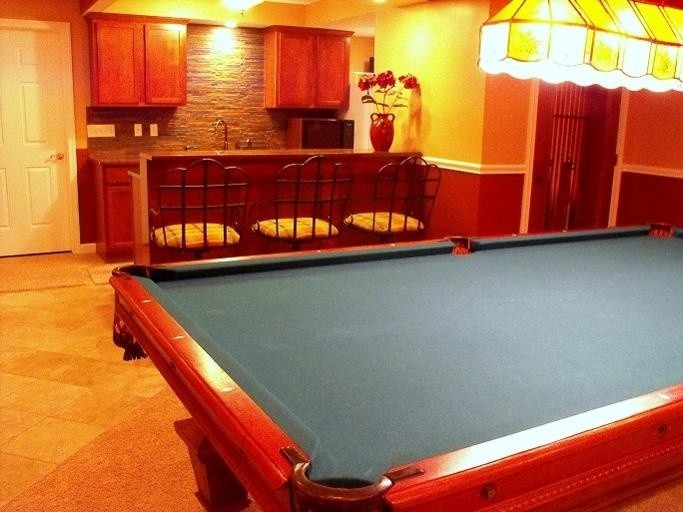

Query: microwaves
[286,117,355,149]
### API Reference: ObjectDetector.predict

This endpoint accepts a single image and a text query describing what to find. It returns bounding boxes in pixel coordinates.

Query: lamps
[475,1,683,92]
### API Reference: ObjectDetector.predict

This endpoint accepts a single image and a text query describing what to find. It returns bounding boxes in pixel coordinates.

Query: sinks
[216,150,241,155]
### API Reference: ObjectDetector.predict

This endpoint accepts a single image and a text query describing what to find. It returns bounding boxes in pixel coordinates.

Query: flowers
[357,71,419,114]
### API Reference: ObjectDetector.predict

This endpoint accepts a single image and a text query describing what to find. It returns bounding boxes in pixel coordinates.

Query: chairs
[146,154,442,261]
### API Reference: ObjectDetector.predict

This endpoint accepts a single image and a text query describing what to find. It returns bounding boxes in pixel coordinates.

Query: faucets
[213,119,228,150]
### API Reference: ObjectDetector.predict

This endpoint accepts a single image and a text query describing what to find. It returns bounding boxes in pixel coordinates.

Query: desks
[108,220,682,512]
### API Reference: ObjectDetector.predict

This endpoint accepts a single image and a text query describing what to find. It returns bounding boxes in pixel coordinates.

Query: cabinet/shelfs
[83,12,191,109]
[261,25,354,110]
[91,164,139,264]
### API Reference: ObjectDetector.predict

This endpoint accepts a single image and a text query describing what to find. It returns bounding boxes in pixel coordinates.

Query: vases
[369,113,396,152]
